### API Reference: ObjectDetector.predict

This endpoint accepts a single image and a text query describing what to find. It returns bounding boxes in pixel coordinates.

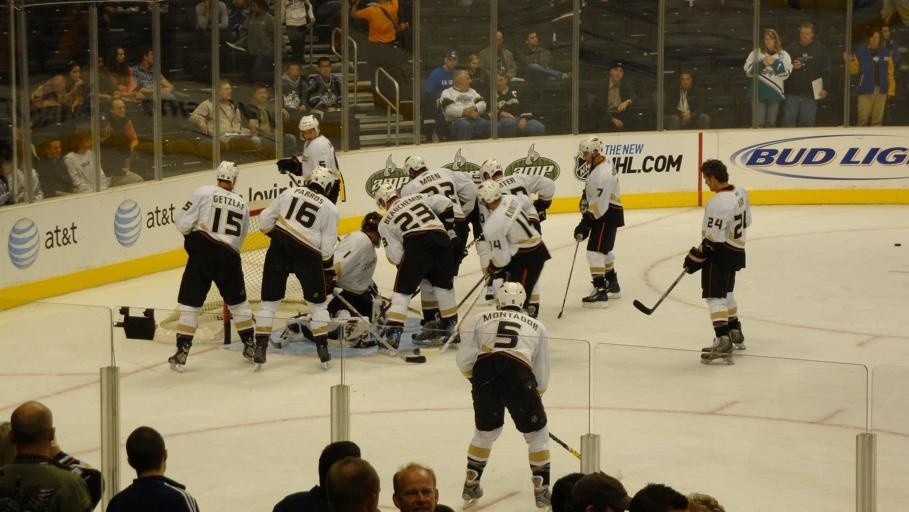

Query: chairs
[1,1,361,208]
[315,0,909,130]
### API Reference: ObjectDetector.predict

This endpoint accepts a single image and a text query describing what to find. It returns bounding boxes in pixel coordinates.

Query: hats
[569,471,632,510]
[446,48,459,58]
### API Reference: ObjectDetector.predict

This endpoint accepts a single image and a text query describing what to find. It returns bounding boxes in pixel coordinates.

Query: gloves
[533,198,552,212]
[579,189,588,215]
[682,246,708,274]
[487,259,506,287]
[573,210,596,242]
[277,156,302,176]
[322,254,338,295]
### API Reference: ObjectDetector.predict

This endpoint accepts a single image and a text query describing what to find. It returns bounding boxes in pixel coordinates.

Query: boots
[168,341,192,365]
[604,270,620,293]
[582,276,608,302]
[462,469,483,500]
[485,276,494,300]
[236,313,461,363]
[531,476,552,508]
[728,321,744,344]
[702,329,733,353]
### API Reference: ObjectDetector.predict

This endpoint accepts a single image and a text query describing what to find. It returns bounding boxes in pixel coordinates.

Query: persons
[166,159,257,365]
[347,1,581,142]
[1,2,342,207]
[106,426,199,512]
[273,440,380,511]
[662,68,710,130]
[551,470,724,511]
[592,60,641,132]
[450,278,562,506]
[378,180,461,349]
[477,178,553,315]
[283,212,388,342]
[392,463,453,511]
[398,153,479,342]
[744,1,908,128]
[681,157,755,358]
[1,400,105,512]
[571,136,625,303]
[251,166,342,364]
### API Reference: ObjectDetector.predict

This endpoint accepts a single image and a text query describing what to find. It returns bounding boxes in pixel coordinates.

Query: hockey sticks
[556,235,582,318]
[251,314,290,348]
[633,268,686,315]
[331,288,426,363]
[421,277,490,353]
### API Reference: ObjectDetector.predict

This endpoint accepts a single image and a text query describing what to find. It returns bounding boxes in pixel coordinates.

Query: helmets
[495,281,527,312]
[403,154,426,177]
[298,114,320,142]
[375,182,399,212]
[361,212,383,249]
[215,160,239,188]
[579,136,604,164]
[479,158,503,182]
[476,179,502,206]
[309,165,339,195]
